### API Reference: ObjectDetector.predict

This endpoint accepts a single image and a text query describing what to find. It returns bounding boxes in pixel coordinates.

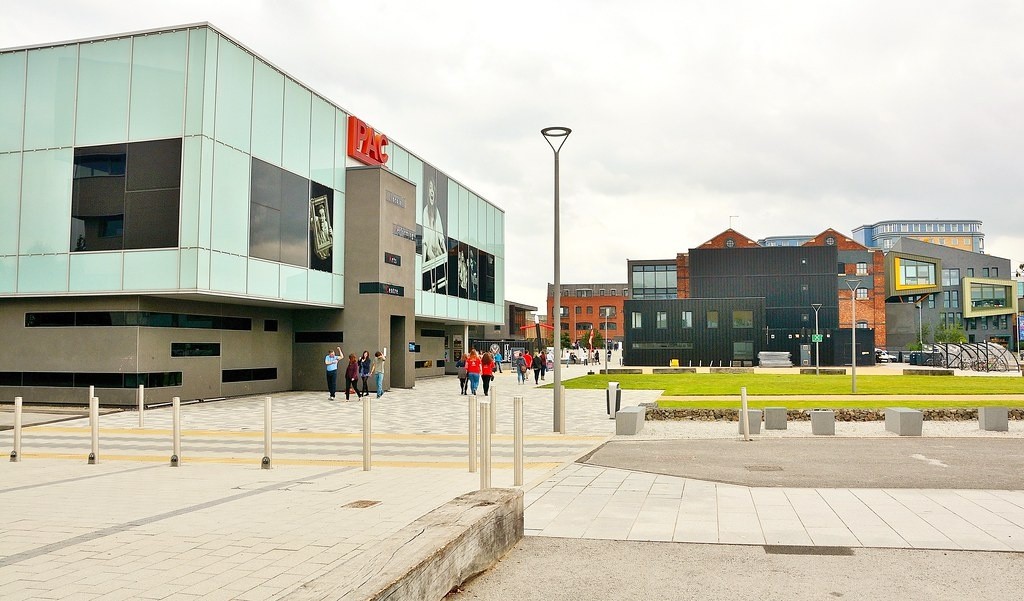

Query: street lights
[844,279,862,392]
[915,305,922,344]
[541,127,573,434]
[812,303,823,375]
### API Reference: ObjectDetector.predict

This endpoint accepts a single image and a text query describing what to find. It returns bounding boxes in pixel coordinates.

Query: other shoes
[377,395,380,398]
[328,396,334,400]
[472,388,477,395]
[364,393,369,396]
[461,391,467,395]
[346,399,349,402]
[518,381,520,384]
[381,393,383,395]
[360,393,364,396]
[485,393,488,395]
[541,375,546,380]
[359,396,362,400]
[522,380,524,383]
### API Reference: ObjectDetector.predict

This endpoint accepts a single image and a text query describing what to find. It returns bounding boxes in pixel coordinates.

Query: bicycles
[957,356,1006,372]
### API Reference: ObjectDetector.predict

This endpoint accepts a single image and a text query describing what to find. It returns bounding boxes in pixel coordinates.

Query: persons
[591,350,601,365]
[310,205,333,270]
[571,352,577,364]
[607,349,612,362]
[325,347,344,400]
[459,251,477,298]
[572,341,579,350]
[455,349,503,396]
[345,353,362,402]
[614,341,618,351]
[516,349,554,385]
[357,350,386,399]
[423,177,446,261]
[583,351,588,365]
[566,351,570,368]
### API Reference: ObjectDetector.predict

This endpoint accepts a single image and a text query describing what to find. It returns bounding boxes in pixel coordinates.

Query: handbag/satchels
[520,365,526,372]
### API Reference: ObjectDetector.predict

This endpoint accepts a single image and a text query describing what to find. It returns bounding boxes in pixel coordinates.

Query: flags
[589,330,594,344]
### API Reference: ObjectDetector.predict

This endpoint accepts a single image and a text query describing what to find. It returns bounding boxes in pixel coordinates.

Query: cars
[874,348,897,363]
